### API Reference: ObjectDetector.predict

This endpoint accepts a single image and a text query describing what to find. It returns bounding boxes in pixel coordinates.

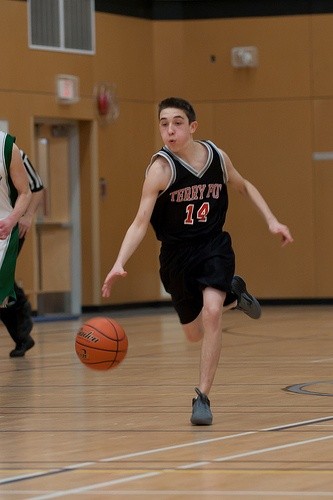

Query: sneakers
[190,386,213,425]
[230,276,261,319]
[10,336,34,356]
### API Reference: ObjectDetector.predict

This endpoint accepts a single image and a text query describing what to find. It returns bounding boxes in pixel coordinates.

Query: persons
[102,98,292,425]
[8,148,44,334]
[0,131,35,357]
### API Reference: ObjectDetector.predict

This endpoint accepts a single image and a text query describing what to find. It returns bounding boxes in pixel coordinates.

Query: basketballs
[75,317,128,370]
